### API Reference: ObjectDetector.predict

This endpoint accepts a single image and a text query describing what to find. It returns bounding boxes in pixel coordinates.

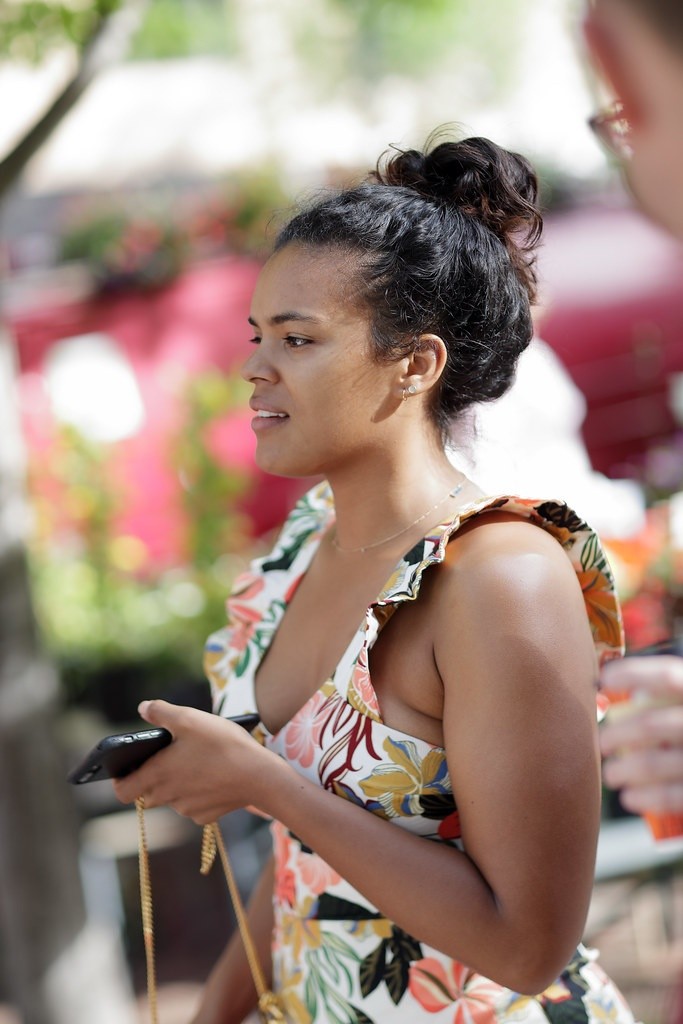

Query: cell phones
[67,713,262,785]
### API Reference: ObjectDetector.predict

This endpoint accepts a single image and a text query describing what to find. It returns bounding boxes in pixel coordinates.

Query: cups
[604,681,683,841]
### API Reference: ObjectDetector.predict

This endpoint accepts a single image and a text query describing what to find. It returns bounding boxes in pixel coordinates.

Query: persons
[658,640,681,655]
[117,136,641,1024]
[583,2,683,812]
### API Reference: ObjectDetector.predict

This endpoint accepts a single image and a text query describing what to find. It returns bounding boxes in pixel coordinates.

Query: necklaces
[330,477,466,552]
